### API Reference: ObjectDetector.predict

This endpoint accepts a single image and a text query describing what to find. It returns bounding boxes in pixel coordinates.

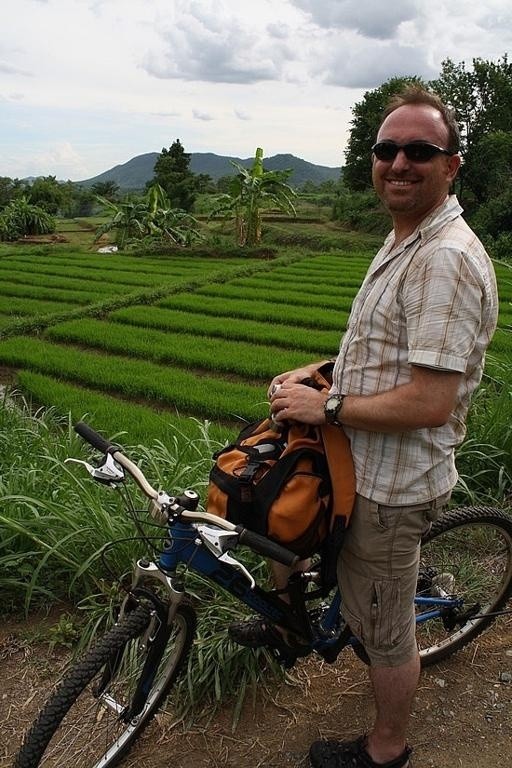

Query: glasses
[371,142,451,161]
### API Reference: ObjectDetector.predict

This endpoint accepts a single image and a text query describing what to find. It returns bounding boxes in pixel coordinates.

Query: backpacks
[207,363,356,550]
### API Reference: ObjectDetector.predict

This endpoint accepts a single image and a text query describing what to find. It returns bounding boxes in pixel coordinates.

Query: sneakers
[310,734,412,768]
[228,621,312,657]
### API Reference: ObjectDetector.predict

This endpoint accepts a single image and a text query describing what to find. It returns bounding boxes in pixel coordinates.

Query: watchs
[323,393,345,428]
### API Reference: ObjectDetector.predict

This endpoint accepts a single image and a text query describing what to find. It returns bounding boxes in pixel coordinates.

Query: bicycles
[10,416,511,768]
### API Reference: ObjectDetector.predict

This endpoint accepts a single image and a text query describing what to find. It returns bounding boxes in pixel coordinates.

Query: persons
[227,82,503,768]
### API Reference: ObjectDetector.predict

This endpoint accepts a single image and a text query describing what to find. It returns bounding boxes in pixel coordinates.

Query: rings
[284,407,288,418]
